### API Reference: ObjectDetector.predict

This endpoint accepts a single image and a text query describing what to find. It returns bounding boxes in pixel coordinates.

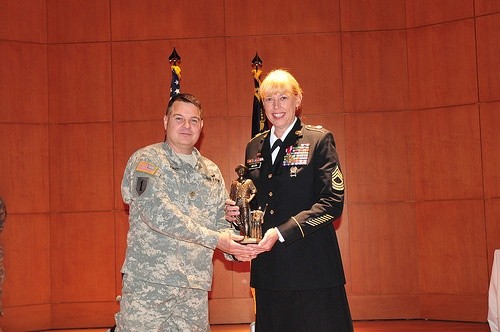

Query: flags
[170,66,180,100]
[250,74,270,315]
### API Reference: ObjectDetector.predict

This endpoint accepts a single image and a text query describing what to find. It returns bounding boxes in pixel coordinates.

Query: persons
[230,164,257,239]
[224,69,354,332]
[113,93,257,332]
